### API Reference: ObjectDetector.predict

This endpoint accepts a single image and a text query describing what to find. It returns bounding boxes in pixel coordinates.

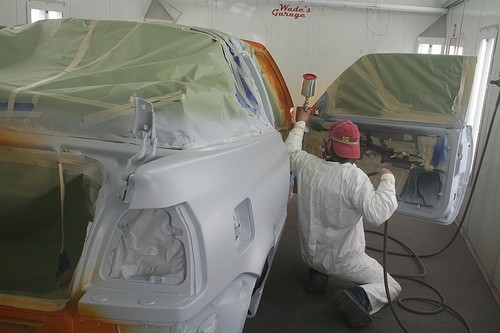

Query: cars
[0,18,492,333]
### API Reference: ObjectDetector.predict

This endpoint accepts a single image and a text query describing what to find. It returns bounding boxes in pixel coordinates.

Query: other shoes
[303,272,324,297]
[333,288,374,329]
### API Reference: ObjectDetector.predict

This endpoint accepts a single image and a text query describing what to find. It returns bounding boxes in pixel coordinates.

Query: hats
[329,119,361,158]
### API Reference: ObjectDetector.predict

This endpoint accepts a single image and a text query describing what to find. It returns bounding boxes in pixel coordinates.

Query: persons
[284,107,401,328]
